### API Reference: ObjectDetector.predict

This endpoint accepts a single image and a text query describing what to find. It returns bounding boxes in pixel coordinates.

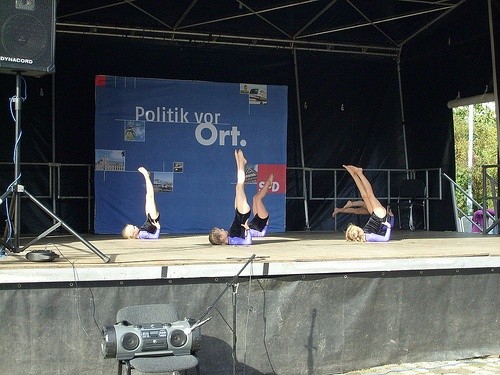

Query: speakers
[0,0,57,78]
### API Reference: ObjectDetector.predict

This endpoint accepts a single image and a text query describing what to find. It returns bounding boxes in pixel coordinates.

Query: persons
[245,174,273,236]
[471,202,495,234]
[333,165,394,242]
[122,166,160,239]
[209,150,251,245]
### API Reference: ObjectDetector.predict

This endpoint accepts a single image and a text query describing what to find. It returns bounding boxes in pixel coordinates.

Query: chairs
[118,304,199,375]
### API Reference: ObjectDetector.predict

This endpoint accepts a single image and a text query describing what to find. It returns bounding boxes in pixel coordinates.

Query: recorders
[102,254,256,360]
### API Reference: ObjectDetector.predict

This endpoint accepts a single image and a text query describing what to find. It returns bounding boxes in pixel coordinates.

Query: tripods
[0,73,111,263]
